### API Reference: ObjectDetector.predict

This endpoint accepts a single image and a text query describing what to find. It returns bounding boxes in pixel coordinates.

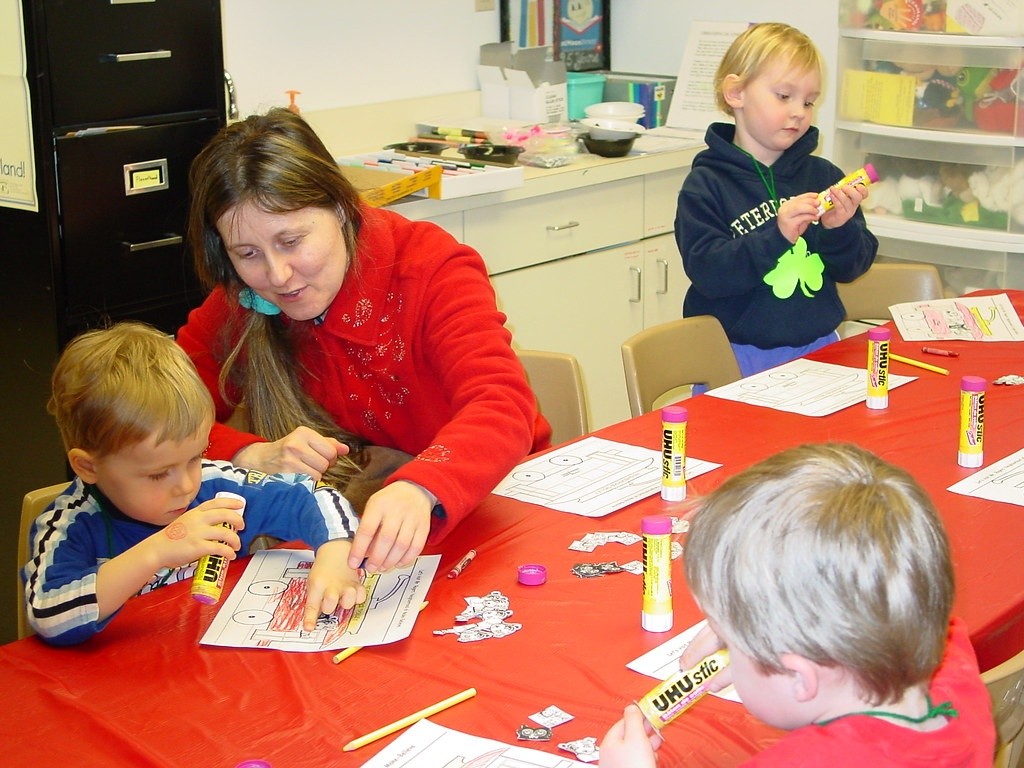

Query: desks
[0,288,1024,768]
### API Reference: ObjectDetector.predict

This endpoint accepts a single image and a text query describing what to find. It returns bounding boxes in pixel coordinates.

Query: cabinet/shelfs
[304,89,710,433]
[23,0,227,480]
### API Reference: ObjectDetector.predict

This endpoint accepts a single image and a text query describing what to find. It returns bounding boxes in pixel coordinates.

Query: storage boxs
[586,70,677,130]
[475,38,569,124]
[816,0,1024,297]
[566,70,608,122]
[334,149,525,200]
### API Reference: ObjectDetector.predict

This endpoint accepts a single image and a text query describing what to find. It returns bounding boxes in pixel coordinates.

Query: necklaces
[817,694,961,726]
[729,142,795,255]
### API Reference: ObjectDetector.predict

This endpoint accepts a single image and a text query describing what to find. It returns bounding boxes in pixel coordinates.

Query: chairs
[14,481,81,643]
[837,263,945,321]
[618,314,742,419]
[514,349,589,446]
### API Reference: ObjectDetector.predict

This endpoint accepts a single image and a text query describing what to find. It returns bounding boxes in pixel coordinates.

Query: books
[510,0,603,71]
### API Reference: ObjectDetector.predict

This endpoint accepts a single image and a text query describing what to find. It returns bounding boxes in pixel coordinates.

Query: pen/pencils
[341,686,478,751]
[350,127,492,176]
[888,354,950,377]
[332,601,430,664]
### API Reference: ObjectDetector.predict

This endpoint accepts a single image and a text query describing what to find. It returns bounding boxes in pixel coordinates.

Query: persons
[173,108,553,574]
[19,323,366,647]
[595,442,997,768]
[674,22,879,397]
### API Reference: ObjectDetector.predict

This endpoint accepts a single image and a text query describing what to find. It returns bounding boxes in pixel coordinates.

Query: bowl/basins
[382,142,449,156]
[457,145,526,166]
[579,117,646,142]
[584,102,646,124]
[577,132,641,158]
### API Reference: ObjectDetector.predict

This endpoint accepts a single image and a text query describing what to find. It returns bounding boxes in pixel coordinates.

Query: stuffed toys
[868,43,999,130]
